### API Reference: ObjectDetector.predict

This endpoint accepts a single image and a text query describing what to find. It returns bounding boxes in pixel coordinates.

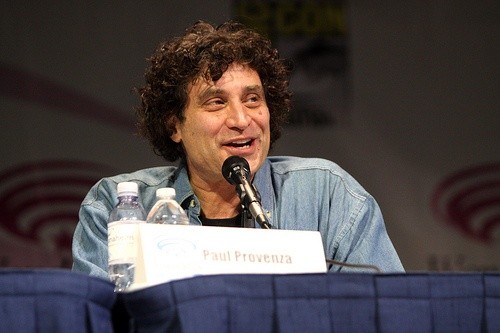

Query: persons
[71,22,406,274]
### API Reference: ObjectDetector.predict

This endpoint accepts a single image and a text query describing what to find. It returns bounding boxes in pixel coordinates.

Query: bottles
[108,181,147,286]
[144,187,190,226]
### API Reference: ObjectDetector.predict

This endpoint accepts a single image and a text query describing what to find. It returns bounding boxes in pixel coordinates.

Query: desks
[0,270,500,333]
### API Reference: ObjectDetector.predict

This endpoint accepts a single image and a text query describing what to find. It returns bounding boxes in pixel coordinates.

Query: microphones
[222,156,272,229]
